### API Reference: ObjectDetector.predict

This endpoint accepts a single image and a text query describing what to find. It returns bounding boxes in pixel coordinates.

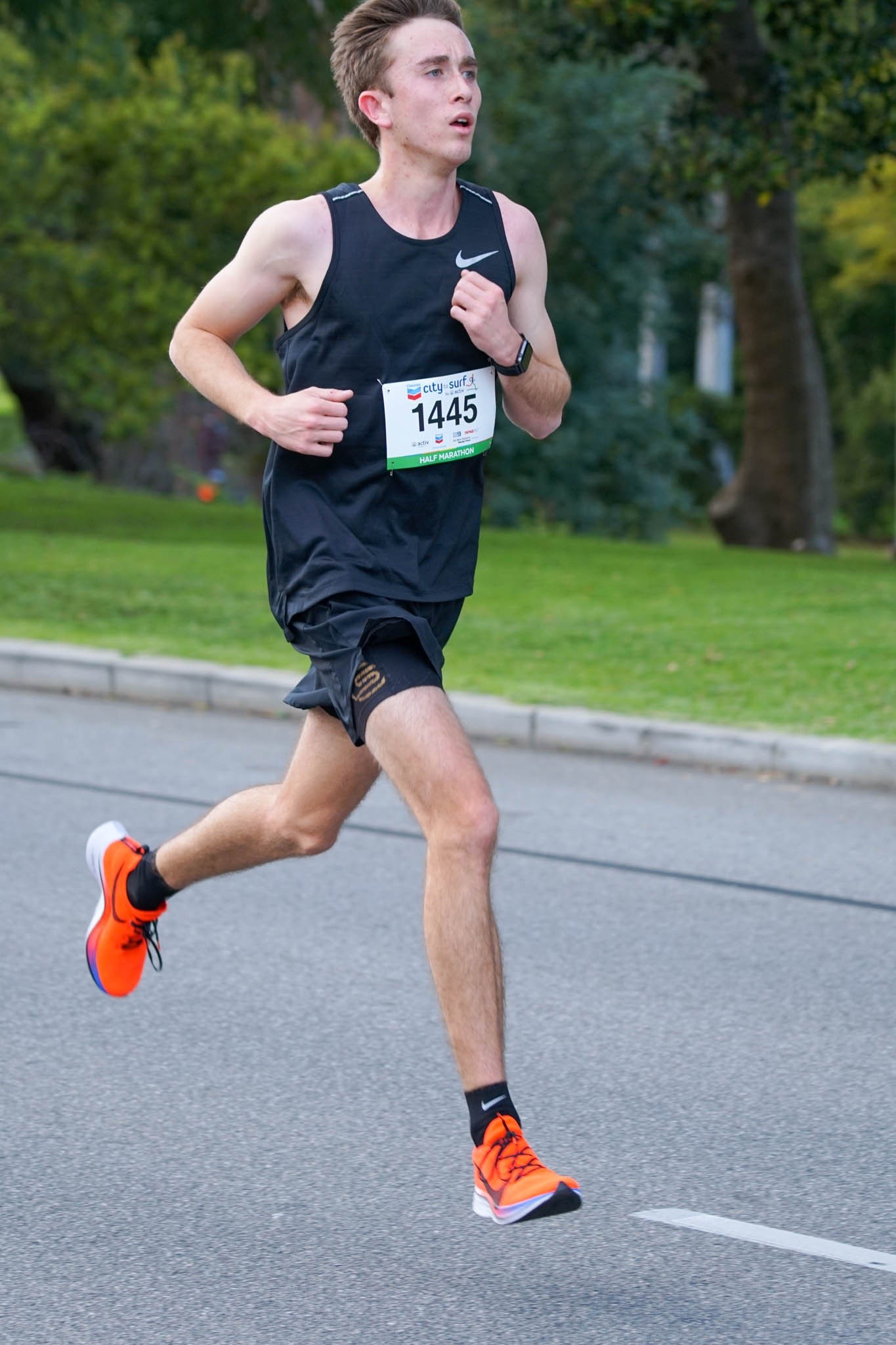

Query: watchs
[488,333,536,363]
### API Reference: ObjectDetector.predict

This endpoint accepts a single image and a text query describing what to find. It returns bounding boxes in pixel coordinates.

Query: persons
[83,0,582,1224]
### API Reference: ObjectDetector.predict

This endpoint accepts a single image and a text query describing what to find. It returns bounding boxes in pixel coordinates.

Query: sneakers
[84,820,170,998]
[471,1113,583,1226]
